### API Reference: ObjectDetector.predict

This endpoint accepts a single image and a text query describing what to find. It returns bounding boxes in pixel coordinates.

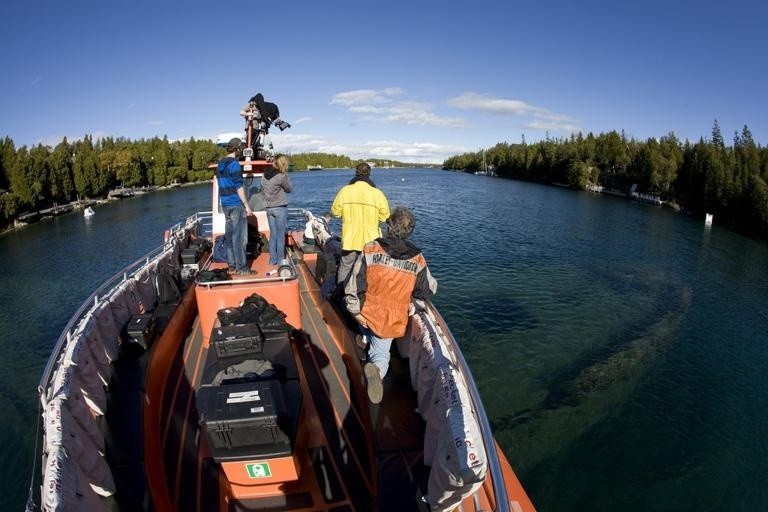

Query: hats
[228,138,246,149]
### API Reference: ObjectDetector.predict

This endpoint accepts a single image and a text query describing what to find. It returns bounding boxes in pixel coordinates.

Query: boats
[26,89,541,512]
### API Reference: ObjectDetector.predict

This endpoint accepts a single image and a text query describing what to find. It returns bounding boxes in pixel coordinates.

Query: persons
[261,153,291,267]
[216,137,257,275]
[341,206,438,406]
[240,101,261,147]
[330,162,392,304]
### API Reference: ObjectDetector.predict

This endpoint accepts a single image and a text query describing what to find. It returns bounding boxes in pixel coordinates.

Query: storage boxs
[196,379,301,449]
[212,323,264,359]
[180,248,198,264]
[126,312,157,351]
[316,252,336,286]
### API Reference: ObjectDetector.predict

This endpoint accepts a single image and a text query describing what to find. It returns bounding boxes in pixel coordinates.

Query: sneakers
[364,362,384,405]
[235,269,257,276]
[355,335,370,351]
